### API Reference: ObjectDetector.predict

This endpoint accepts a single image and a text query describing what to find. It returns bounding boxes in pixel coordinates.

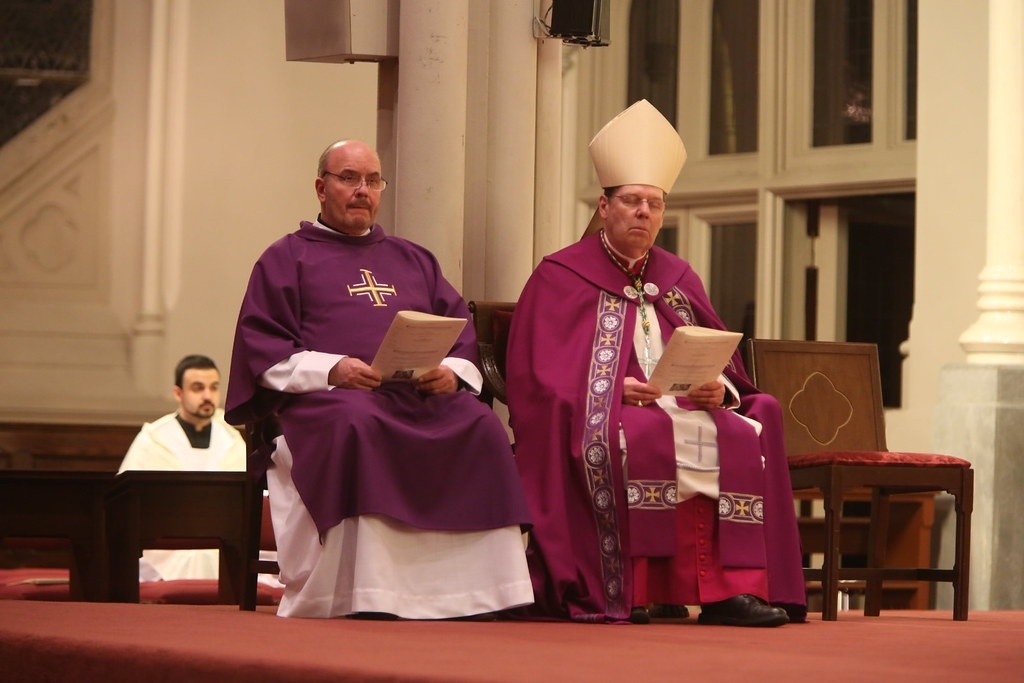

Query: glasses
[606,193,666,216]
[321,171,388,192]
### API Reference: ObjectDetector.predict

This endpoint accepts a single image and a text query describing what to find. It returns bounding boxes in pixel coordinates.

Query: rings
[638,400,644,407]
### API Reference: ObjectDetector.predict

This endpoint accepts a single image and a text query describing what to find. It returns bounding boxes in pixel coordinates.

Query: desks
[791,483,942,611]
[0,469,247,606]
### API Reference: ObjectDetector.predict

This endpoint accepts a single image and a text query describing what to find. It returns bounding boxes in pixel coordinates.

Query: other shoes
[648,600,689,617]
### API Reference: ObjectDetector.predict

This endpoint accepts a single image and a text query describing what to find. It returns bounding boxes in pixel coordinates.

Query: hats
[588,98,688,195]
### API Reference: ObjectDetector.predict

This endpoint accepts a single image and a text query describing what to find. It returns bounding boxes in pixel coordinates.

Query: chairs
[747,338,974,621]
[237,299,519,611]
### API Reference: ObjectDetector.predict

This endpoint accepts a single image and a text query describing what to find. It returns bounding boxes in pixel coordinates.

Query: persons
[224,138,534,621]
[117,354,247,583]
[505,100,809,626]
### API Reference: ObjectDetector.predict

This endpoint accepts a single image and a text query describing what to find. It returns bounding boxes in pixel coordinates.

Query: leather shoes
[624,604,650,625]
[697,589,790,628]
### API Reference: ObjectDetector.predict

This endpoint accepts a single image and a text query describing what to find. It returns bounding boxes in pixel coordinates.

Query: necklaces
[599,228,658,379]
